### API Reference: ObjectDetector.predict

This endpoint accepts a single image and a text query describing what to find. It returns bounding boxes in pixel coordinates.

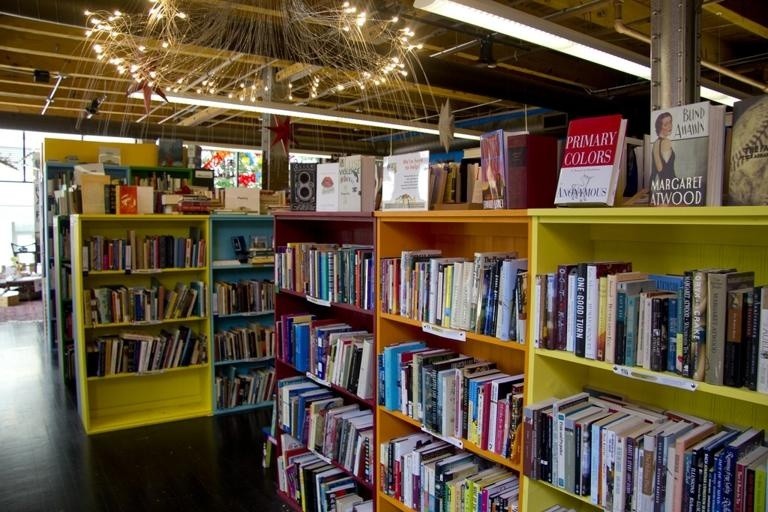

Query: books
[82,278,205,325]
[273,241,376,312]
[82,234,206,272]
[86,327,207,381]
[50,226,74,377]
[533,261,768,397]
[379,246,528,345]
[375,431,518,512]
[47,174,285,217]
[520,384,767,512]
[726,98,767,205]
[209,246,274,410]
[431,161,460,204]
[289,163,337,212]
[274,313,377,400]
[376,339,520,460]
[480,129,509,207]
[504,134,552,206]
[651,100,724,205]
[337,156,375,211]
[383,151,432,210]
[274,376,377,512]
[554,115,626,205]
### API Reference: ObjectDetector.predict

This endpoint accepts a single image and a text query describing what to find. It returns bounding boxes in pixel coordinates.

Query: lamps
[413,0,751,111]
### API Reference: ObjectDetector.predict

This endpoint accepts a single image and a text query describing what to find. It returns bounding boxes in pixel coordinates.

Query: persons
[652,110,679,181]
